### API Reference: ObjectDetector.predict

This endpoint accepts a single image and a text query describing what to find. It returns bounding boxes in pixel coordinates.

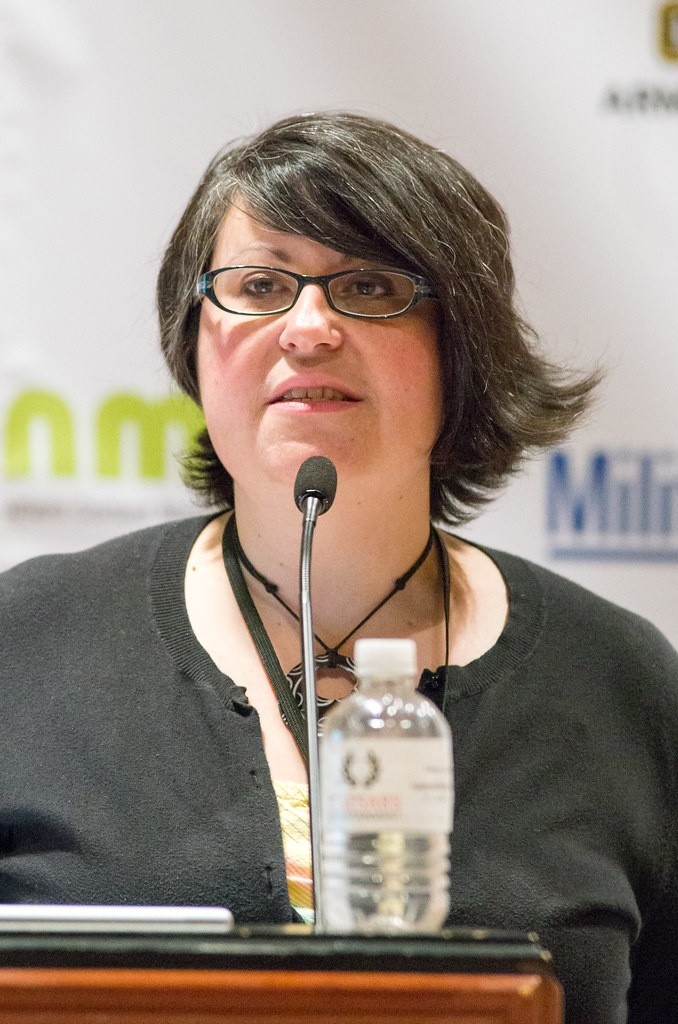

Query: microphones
[294,456,338,937]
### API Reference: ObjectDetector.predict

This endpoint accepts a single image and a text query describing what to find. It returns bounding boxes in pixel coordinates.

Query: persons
[0,112,678,1024]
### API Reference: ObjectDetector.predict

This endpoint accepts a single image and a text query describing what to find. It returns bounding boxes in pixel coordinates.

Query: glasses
[193,262,434,320]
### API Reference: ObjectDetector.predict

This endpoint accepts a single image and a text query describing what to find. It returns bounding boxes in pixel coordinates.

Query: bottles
[318,639,454,935]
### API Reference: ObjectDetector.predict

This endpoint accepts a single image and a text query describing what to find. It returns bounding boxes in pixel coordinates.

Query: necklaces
[227,516,435,739]
